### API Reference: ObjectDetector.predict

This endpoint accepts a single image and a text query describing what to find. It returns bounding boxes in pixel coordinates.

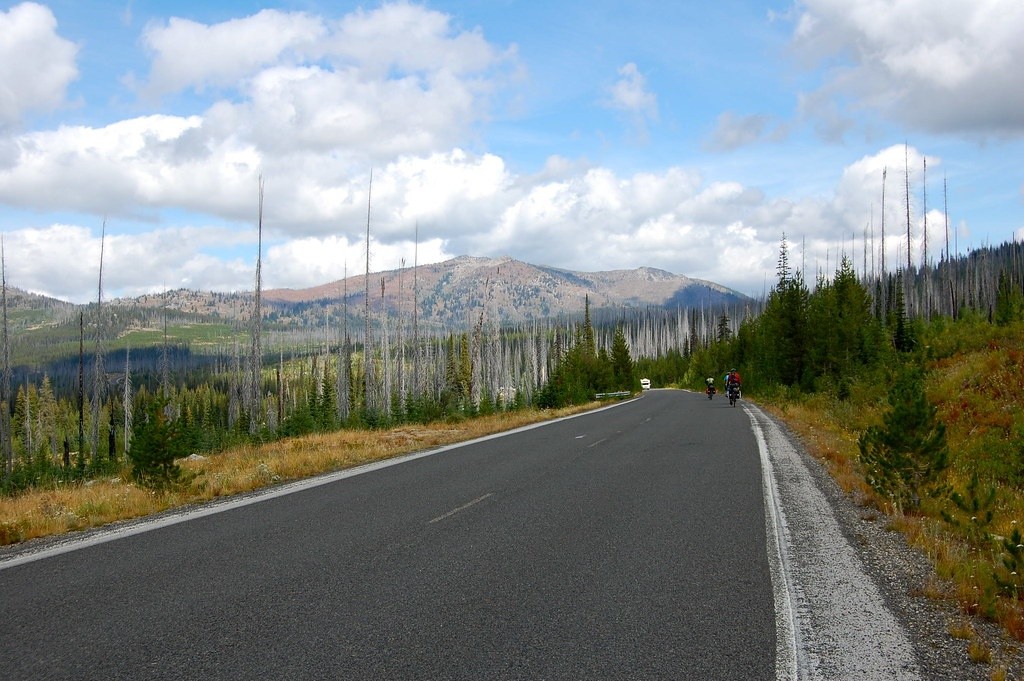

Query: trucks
[640,379,650,390]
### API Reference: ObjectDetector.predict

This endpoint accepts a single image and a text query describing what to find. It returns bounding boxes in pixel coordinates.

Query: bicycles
[708,384,716,399]
[729,383,739,407]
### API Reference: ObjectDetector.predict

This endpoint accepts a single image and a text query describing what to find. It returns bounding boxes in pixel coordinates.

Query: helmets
[730,368,736,371]
[727,372,730,374]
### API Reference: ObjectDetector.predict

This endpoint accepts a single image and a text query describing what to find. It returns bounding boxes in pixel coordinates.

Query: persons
[706,374,714,398]
[724,369,741,407]
